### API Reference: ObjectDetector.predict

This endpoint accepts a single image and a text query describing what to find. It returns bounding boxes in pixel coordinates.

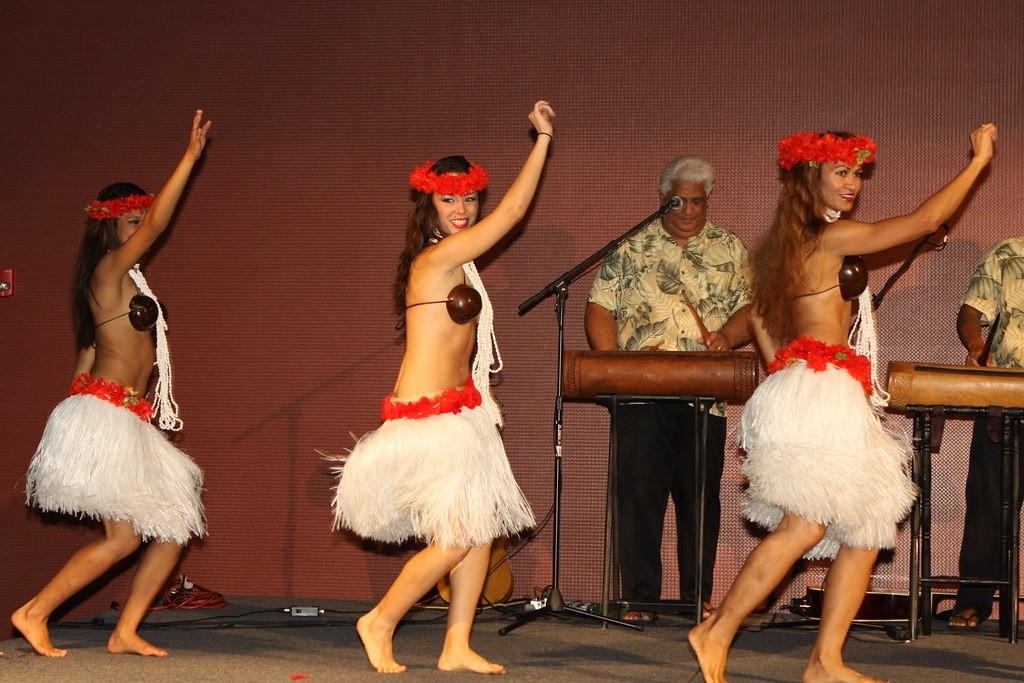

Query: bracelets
[537,132,553,138]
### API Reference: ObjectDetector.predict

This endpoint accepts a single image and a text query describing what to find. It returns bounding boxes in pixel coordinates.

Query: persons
[581,155,757,625]
[332,99,556,675]
[688,120,997,683]
[7,109,211,660]
[948,233,1024,630]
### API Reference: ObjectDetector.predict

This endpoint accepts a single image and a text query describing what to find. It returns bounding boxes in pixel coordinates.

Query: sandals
[947,613,980,631]
[621,609,658,624]
[703,601,716,619]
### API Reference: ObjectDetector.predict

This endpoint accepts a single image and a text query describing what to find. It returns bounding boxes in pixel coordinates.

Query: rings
[717,346,722,350]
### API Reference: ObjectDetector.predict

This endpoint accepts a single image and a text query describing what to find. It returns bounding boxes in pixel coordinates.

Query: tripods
[498,204,675,637]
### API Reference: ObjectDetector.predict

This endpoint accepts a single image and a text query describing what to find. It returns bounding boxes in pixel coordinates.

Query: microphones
[669,195,684,210]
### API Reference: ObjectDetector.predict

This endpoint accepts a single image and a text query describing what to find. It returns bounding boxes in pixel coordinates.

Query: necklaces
[820,206,891,409]
[428,235,506,428]
[127,264,185,432]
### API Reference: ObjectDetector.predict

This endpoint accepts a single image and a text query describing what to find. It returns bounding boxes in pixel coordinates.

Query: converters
[291,605,319,617]
